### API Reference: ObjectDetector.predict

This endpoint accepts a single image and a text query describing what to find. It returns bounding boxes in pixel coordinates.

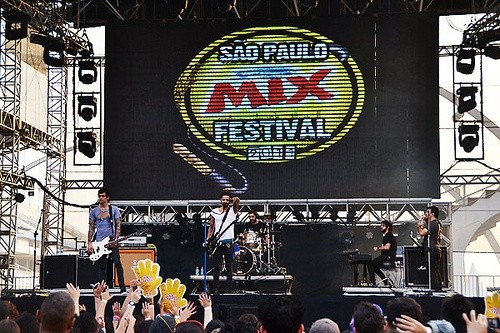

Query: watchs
[128,301,136,306]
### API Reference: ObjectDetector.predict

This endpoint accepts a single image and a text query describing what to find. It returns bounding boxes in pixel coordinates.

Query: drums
[242,230,259,249]
[238,232,243,245]
[224,245,257,276]
[249,237,264,257]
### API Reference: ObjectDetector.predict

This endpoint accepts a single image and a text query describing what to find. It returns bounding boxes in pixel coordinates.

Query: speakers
[42,255,112,289]
[115,249,154,287]
[404,246,448,288]
[219,304,258,325]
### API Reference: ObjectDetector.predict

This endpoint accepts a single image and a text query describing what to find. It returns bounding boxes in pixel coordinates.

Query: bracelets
[377,247,379,250]
[113,315,121,321]
[94,296,102,298]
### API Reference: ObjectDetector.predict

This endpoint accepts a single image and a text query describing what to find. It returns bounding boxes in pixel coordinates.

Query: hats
[309,318,340,333]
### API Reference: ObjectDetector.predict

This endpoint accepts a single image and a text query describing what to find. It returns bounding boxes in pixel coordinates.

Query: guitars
[86,226,151,262]
[205,219,237,258]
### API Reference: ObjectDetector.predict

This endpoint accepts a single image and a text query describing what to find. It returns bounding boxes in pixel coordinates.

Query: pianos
[339,248,373,287]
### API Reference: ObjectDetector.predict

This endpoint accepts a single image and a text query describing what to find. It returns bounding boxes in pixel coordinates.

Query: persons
[203,192,240,294]
[237,210,271,274]
[417,206,443,291]
[0,280,500,333]
[88,189,127,295]
[368,220,397,288]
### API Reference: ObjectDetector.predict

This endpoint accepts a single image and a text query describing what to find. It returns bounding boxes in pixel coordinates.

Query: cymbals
[256,215,277,220]
[270,230,282,233]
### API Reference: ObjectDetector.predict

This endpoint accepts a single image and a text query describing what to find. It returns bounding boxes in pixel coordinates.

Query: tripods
[256,238,275,273]
